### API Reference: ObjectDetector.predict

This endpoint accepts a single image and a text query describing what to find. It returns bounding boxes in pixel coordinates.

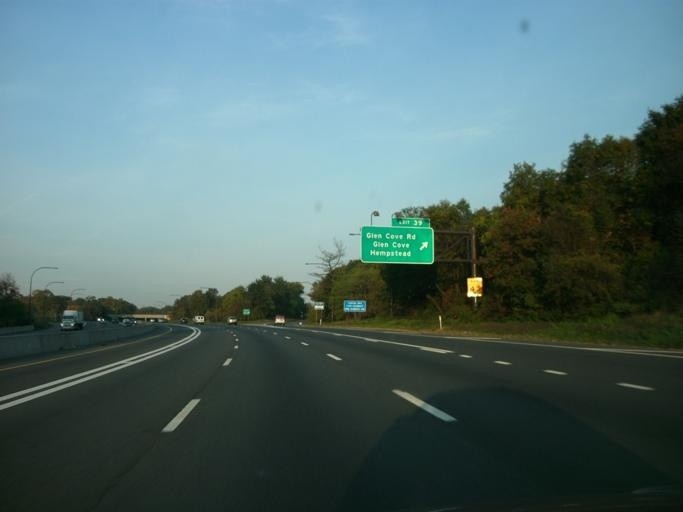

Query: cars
[93,316,168,328]
[180,315,206,325]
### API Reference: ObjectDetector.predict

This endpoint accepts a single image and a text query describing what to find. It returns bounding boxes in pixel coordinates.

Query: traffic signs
[359,215,435,266]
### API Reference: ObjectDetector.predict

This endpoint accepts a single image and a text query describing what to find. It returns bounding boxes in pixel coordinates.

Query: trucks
[61,310,84,329]
[274,315,286,326]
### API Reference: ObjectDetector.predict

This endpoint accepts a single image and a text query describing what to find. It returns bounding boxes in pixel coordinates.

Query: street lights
[29,266,87,328]
[156,287,218,322]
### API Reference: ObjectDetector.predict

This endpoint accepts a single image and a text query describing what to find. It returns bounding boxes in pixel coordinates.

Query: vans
[228,316,237,325]
